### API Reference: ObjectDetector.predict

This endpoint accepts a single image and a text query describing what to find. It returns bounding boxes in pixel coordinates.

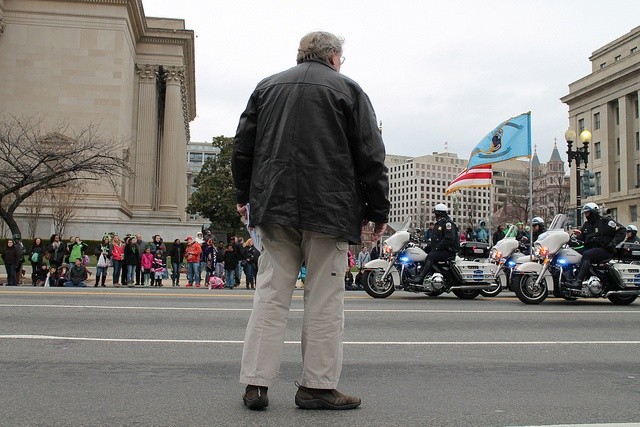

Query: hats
[226,243,234,247]
[156,250,163,254]
[184,236,192,241]
[102,233,109,240]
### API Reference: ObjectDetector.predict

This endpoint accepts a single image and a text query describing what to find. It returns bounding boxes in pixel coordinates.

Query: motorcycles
[361,213,497,299]
[510,213,640,304]
[480,223,528,298]
[565,128,592,230]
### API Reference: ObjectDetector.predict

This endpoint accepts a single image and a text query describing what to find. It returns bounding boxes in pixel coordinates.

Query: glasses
[335,54,344,62]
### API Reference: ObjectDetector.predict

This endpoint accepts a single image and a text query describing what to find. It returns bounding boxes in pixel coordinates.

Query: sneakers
[240,384,269,410]
[195,284,200,287]
[294,383,361,410]
[186,283,192,286]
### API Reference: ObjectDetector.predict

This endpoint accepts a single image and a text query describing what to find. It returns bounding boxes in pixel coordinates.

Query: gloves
[607,242,616,253]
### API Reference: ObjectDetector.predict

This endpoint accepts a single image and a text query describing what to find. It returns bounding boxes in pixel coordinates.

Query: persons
[465,223,474,241]
[3,230,261,289]
[494,225,505,245]
[474,220,489,243]
[516,221,530,252]
[348,249,355,267]
[358,246,372,272]
[407,204,459,283]
[232,33,390,405]
[356,268,364,289]
[425,222,435,239]
[346,265,358,291]
[564,203,626,290]
[372,240,384,260]
[627,224,639,249]
[521,217,548,255]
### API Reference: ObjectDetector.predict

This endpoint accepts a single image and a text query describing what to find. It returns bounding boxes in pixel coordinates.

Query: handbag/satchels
[357,253,368,270]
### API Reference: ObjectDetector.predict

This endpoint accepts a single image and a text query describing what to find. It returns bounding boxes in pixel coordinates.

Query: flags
[445,163,492,200]
[466,112,531,170]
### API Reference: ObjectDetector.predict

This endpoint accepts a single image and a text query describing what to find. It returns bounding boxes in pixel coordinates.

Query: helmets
[625,224,637,231]
[13,234,21,239]
[581,203,600,214]
[432,204,448,214]
[532,217,545,225]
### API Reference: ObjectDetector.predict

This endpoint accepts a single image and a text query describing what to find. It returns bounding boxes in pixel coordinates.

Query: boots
[565,260,591,289]
[406,259,433,285]
